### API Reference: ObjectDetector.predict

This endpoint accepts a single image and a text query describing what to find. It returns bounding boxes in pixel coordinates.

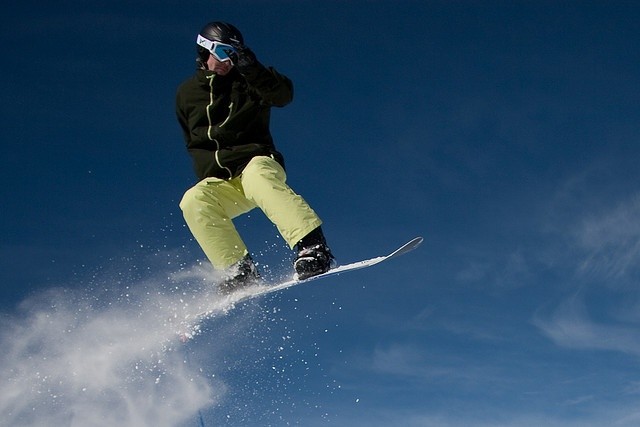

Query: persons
[178,22,335,294]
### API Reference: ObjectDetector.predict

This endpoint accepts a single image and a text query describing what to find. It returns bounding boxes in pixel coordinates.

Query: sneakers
[293,225,334,281]
[217,253,258,296]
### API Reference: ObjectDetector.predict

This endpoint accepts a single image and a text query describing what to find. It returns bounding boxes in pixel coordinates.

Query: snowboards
[240,236,423,302]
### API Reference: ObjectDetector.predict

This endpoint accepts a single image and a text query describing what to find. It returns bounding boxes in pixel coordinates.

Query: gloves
[227,43,274,90]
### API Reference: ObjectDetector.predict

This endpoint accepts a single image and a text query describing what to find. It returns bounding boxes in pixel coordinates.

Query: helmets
[195,22,244,62]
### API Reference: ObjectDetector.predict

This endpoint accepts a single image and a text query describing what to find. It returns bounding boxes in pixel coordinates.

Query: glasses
[196,34,234,66]
